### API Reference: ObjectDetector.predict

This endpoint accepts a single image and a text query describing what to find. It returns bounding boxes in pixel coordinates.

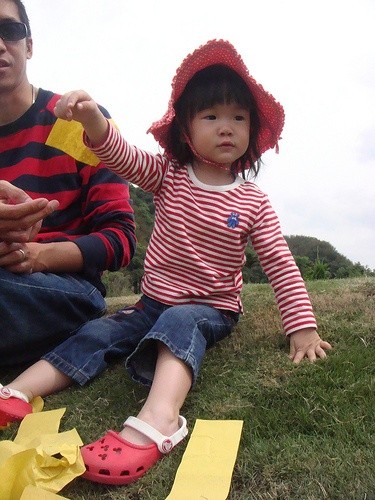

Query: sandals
[0,382,32,426]
[79,415,189,484]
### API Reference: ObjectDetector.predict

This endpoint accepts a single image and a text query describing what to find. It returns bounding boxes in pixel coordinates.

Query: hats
[145,39,285,174]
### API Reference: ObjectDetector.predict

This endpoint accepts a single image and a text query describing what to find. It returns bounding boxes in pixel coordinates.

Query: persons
[0,39,332,486]
[0,0,137,370]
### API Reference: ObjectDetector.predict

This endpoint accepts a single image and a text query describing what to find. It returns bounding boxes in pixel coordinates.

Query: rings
[19,249,25,259]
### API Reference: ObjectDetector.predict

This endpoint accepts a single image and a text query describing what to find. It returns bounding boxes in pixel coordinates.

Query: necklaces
[29,84,35,105]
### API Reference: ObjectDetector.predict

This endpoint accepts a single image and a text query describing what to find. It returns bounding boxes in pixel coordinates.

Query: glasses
[0,22,28,41]
